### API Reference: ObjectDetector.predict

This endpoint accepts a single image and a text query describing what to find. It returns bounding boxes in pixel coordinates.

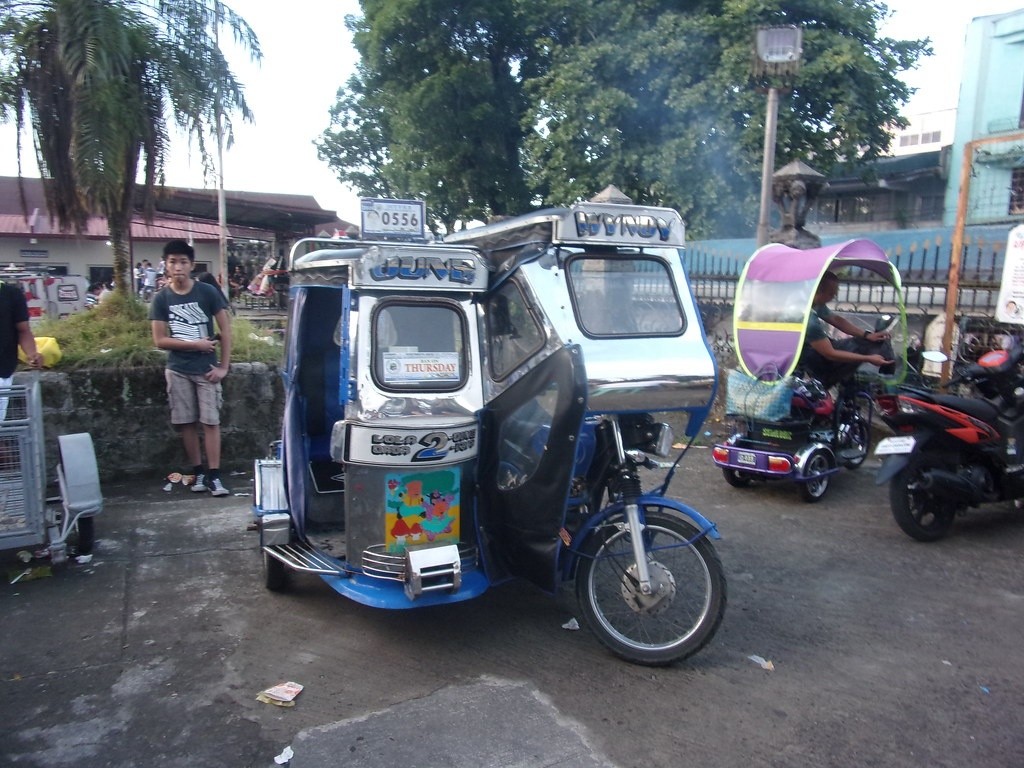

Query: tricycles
[712,238,908,500]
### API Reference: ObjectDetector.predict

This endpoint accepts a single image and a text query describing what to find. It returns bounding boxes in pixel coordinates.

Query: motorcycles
[247,191,732,669]
[878,328,1024,542]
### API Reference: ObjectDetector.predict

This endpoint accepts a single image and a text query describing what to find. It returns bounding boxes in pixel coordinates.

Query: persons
[197,250,286,303]
[86,281,113,306]
[135,257,171,299]
[798,269,895,387]
[0,280,43,427]
[148,241,231,496]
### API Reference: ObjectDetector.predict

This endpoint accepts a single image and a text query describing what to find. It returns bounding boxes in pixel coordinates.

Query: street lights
[752,24,804,250]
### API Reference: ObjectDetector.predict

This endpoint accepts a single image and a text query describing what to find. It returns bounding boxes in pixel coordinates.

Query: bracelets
[864,329,871,336]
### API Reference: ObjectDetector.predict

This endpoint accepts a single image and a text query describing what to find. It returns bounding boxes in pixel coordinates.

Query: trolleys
[0,358,104,581]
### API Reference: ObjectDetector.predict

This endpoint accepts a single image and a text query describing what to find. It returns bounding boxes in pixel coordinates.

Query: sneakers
[206,475,230,496]
[190,469,208,493]
